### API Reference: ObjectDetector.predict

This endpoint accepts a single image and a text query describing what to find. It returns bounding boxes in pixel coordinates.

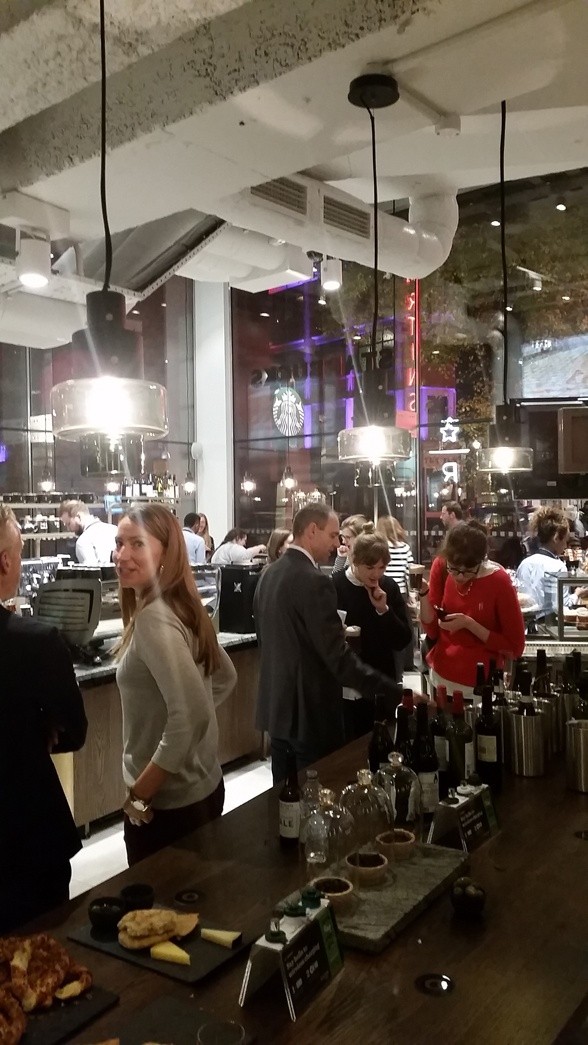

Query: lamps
[474,102,534,473]
[14,235,51,288]
[533,277,543,292]
[241,472,258,493]
[321,252,343,293]
[338,74,412,466]
[183,279,195,494]
[49,0,170,441]
[280,385,297,490]
[37,404,57,492]
[318,291,326,306]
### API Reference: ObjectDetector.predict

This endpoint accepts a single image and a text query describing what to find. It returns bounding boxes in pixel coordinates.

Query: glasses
[444,558,483,580]
[341,535,355,542]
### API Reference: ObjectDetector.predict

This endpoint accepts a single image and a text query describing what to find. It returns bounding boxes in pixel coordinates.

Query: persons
[111,502,238,870]
[330,521,418,687]
[0,501,88,927]
[61,496,588,599]
[417,519,524,707]
[253,503,427,787]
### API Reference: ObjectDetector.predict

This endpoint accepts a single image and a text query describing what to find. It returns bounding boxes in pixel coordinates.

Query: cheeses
[150,941,190,965]
[200,928,243,949]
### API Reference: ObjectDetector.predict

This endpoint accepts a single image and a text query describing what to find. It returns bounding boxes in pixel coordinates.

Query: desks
[0,720,588,1045]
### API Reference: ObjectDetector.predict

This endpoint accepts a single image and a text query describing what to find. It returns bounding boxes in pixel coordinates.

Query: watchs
[130,787,153,813]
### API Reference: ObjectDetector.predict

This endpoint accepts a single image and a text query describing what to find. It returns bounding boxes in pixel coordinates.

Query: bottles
[121,469,180,504]
[277,751,302,854]
[488,649,588,720]
[367,685,474,827]
[298,751,420,876]
[474,684,504,785]
[472,662,487,707]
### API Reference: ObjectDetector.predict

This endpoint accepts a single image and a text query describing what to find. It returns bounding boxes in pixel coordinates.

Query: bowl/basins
[344,852,388,884]
[374,828,417,863]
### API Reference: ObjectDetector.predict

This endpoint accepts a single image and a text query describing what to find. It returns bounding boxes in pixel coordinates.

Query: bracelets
[419,587,430,597]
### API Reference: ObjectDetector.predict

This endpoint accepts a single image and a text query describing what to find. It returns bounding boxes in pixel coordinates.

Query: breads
[0,934,94,1045]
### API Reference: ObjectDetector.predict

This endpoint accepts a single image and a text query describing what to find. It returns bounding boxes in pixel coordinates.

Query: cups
[407,564,425,592]
[337,610,347,624]
[4,493,94,504]
[195,1018,246,1045]
[309,876,354,910]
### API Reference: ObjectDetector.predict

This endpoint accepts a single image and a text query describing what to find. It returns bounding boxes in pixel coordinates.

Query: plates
[65,903,258,986]
[16,986,121,1045]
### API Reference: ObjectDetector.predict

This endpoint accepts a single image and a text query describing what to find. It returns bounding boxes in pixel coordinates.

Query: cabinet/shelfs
[0,503,105,558]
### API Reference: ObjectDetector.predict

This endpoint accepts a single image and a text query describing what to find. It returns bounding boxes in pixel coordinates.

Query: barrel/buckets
[463,682,588,794]
[19,514,68,534]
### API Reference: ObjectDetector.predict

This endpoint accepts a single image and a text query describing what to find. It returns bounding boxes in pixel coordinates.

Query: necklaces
[455,578,474,595]
